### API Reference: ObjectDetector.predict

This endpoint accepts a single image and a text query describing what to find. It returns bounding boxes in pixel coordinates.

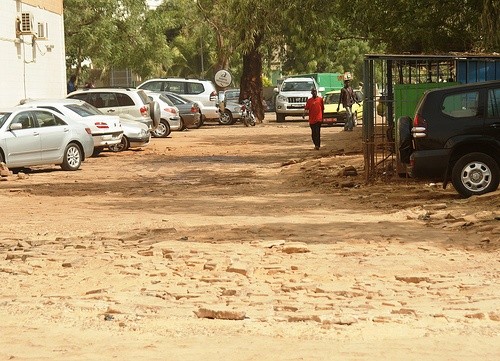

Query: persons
[67,75,77,94]
[302,89,324,150]
[337,80,361,131]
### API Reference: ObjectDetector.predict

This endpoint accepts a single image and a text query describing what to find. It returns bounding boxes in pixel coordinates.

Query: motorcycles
[240,97,256,127]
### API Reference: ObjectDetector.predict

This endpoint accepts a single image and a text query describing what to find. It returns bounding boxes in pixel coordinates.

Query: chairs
[92,97,102,107]
[22,117,34,128]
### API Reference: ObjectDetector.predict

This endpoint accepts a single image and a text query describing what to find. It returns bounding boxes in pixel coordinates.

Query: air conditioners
[37,21,48,39]
[21,11,33,32]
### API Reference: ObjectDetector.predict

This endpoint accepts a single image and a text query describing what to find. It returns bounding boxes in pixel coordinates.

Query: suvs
[273,75,325,123]
[393,81,500,196]
[135,78,219,127]
[66,87,161,132]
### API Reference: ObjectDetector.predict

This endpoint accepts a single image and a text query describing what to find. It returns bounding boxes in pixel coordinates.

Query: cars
[219,102,245,124]
[104,116,152,151]
[147,93,181,137]
[0,105,94,171]
[324,90,365,127]
[152,91,201,130]
[19,98,123,159]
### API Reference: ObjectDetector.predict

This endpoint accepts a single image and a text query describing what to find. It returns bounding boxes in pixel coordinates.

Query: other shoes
[315,146,319,150]
